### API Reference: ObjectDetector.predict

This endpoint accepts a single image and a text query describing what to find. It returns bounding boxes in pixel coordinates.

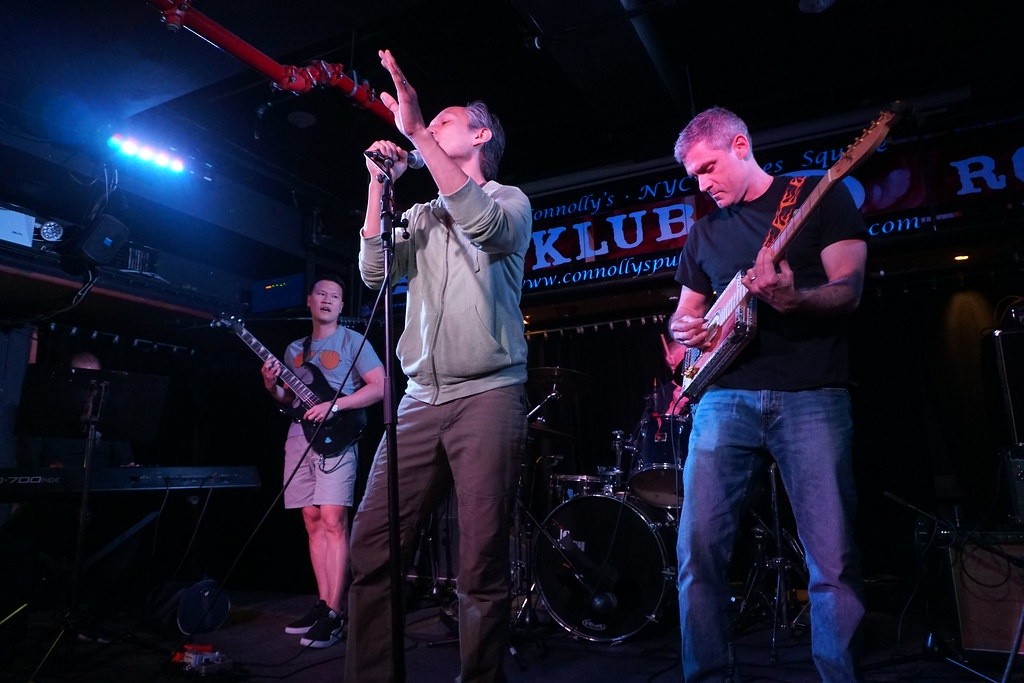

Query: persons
[261,271,388,650]
[341,49,542,683]
[662,105,872,683]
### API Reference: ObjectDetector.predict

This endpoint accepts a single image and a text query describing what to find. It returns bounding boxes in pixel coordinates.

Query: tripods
[734,457,820,665]
[0,323,195,683]
[427,390,564,671]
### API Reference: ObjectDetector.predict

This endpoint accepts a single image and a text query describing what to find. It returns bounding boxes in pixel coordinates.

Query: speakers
[73,215,130,267]
[981,322,1023,447]
[947,524,1024,670]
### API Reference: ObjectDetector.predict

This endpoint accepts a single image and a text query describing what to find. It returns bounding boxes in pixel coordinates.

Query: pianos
[1,465,262,502]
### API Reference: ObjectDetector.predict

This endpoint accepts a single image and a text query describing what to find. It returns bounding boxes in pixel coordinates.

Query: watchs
[330,400,339,417]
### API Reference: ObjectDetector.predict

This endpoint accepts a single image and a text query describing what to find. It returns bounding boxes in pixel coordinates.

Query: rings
[750,275,757,283]
[403,80,406,84]
[313,417,319,423]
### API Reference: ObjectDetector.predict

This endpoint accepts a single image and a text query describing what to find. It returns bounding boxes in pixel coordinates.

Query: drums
[627,414,692,509]
[531,490,682,645]
[549,474,624,513]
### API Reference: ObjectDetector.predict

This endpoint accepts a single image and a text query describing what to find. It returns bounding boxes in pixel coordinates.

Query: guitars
[210,311,368,458]
[679,109,896,400]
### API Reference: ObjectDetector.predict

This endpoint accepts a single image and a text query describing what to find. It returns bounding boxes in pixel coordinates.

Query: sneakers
[284,597,329,634]
[300,604,347,648]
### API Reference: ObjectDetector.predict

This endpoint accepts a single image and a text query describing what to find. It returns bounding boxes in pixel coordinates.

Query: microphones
[366,148,425,169]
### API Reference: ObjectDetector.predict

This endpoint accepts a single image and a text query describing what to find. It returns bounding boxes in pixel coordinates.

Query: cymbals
[529,416,578,443]
[527,367,599,403]
